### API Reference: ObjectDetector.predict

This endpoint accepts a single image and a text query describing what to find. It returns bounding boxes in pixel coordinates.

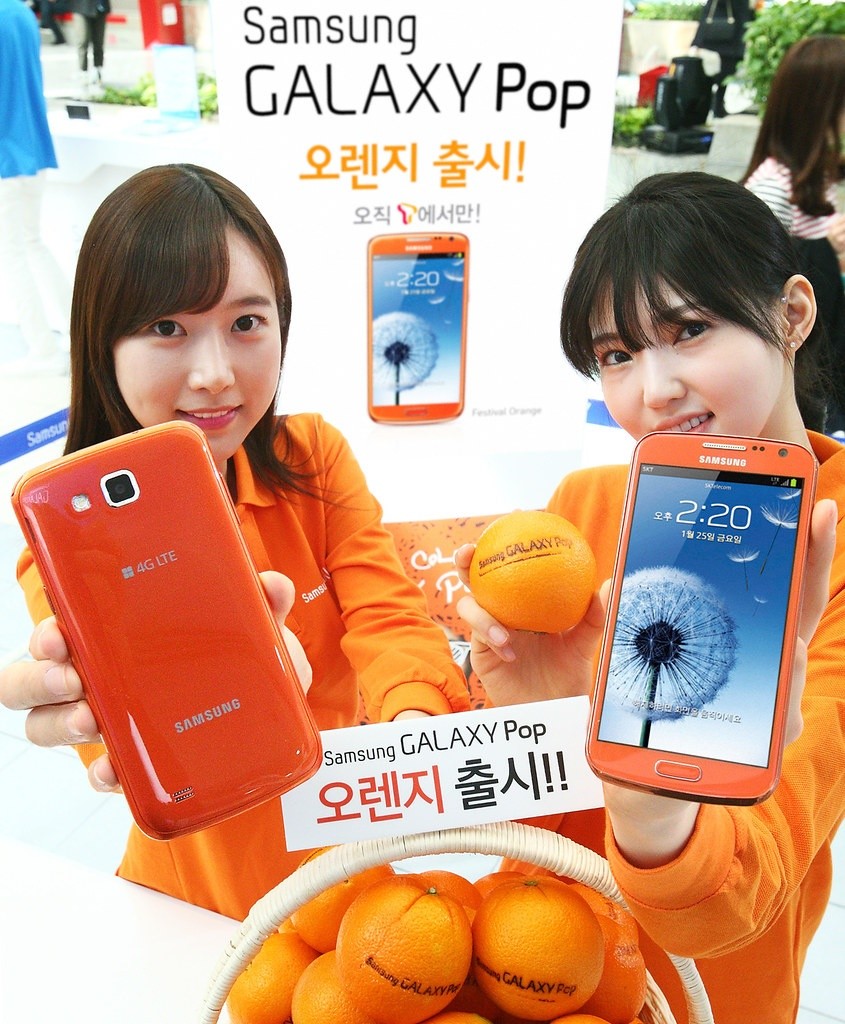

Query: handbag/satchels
[703,15,738,42]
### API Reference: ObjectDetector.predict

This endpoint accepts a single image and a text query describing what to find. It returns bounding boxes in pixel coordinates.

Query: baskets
[199,822,715,1024]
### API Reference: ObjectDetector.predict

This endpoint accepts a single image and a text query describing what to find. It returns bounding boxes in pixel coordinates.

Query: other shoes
[713,106,728,118]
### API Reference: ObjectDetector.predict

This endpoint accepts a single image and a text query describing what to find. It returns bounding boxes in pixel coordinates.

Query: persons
[739,35,844,439]
[692,0,752,117]
[1,161,475,922]
[456,170,844,1024]
[27,0,110,84]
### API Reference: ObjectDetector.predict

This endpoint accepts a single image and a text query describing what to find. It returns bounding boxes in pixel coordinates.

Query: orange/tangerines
[469,510,596,634]
[221,844,647,1024]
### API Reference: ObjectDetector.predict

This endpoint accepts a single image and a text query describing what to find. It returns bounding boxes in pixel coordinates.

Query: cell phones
[366,232,470,426]
[7,420,324,838]
[585,431,817,806]
